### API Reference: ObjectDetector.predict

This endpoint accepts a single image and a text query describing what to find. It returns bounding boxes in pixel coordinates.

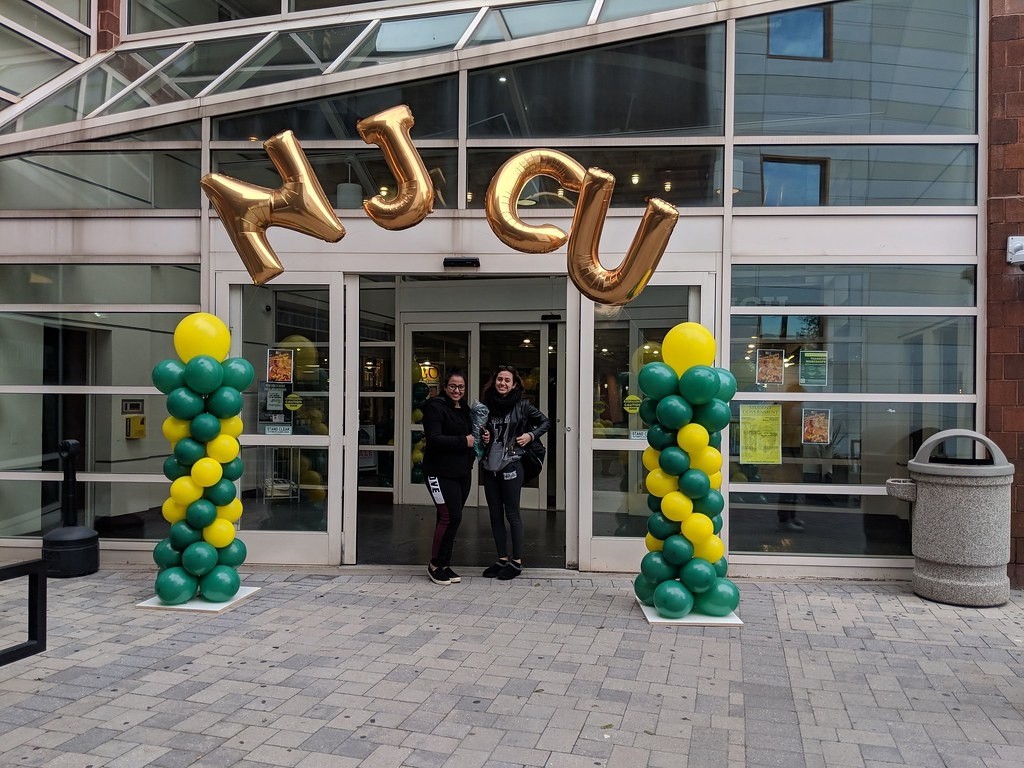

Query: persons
[420,369,491,586]
[474,364,552,582]
[771,383,809,535]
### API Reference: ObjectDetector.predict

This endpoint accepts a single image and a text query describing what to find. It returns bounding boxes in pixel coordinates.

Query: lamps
[548,346,557,354]
[600,349,612,356]
[518,338,535,347]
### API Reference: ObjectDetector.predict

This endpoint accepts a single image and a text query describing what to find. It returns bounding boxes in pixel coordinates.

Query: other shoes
[443,567,461,583]
[499,560,522,579]
[483,559,509,578]
[426,563,451,586]
[777,516,805,533]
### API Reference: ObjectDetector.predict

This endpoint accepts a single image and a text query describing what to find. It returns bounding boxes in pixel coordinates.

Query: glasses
[496,365,515,373]
[446,384,465,390]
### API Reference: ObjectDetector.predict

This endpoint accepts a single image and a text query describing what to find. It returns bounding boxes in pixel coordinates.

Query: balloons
[632,321,742,621]
[729,357,769,522]
[388,360,431,484]
[150,310,257,607]
[613,340,664,538]
[256,333,329,531]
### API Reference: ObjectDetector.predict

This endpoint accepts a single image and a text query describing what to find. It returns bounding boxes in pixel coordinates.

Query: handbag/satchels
[517,400,546,480]
